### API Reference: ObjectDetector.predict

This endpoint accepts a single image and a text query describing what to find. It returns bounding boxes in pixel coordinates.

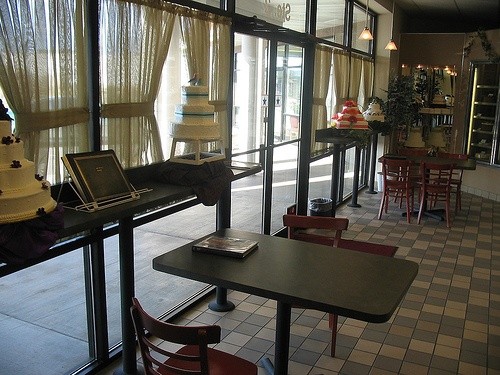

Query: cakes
[363,98,385,122]
[0,99,55,220]
[331,101,368,129]
[172,73,220,139]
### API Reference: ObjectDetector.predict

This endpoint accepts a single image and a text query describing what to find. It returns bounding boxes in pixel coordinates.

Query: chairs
[282,216,349,359]
[376,148,469,227]
[130,296,258,375]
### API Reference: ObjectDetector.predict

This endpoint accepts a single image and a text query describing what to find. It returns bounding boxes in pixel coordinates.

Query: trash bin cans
[309,198,332,229]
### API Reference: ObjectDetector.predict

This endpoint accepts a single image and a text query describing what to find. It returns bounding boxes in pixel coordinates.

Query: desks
[314,128,379,208]
[0,164,263,375]
[379,155,476,222]
[153,229,418,375]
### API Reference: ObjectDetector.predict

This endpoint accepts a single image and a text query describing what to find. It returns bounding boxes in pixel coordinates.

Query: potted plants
[374,72,426,181]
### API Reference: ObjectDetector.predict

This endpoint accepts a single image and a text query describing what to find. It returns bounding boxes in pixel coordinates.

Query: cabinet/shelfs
[400,63,454,136]
[464,60,500,170]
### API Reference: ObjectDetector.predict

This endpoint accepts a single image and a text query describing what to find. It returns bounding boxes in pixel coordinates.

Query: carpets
[291,232,399,258]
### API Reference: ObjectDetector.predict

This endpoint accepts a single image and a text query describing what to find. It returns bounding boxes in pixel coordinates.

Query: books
[192,236,259,258]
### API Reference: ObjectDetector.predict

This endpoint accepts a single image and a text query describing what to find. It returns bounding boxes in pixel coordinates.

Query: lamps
[385,0,397,50]
[358,0,374,40]
